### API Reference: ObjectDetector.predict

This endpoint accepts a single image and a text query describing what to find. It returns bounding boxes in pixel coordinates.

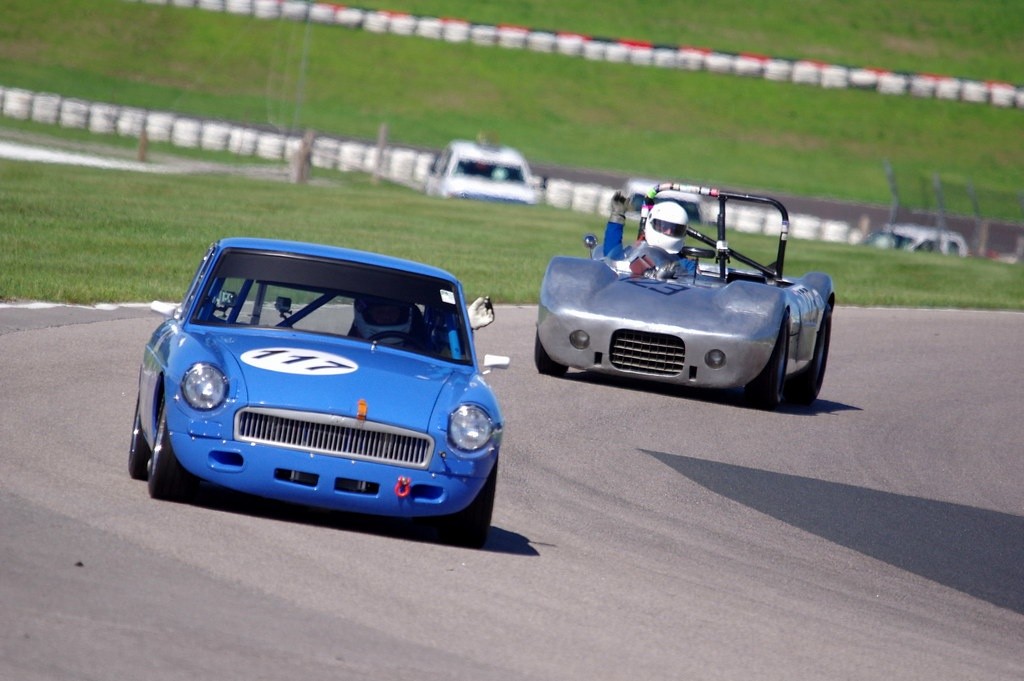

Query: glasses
[656,221,683,236]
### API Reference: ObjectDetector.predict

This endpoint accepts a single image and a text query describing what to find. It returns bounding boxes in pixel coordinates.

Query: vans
[424,139,535,206]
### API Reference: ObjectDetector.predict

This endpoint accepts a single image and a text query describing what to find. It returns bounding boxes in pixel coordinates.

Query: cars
[626,181,708,227]
[129,238,511,548]
[855,226,969,258]
[531,184,840,412]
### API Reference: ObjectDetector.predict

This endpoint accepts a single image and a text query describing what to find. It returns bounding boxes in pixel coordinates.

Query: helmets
[353,293,412,343]
[645,201,689,255]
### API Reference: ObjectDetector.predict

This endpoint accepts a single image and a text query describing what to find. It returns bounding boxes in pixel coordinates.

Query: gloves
[609,190,631,225]
[467,295,494,330]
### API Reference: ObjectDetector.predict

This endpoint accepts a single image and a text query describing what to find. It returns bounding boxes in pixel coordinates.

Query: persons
[602,190,700,280]
[353,294,496,344]
